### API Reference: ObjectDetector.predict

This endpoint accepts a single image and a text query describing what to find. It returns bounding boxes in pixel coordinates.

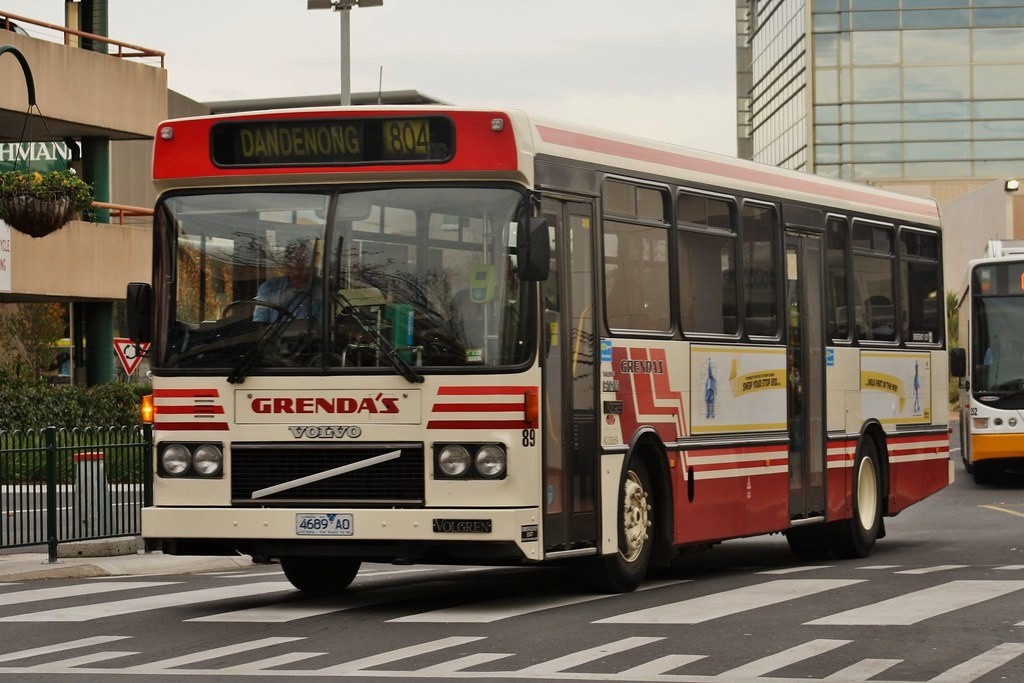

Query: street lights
[1001,177,1019,240]
[308,0,384,106]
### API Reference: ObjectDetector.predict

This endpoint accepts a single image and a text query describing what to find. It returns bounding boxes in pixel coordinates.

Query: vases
[1,188,76,239]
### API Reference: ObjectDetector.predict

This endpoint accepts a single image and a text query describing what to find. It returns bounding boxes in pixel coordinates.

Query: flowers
[0,167,97,224]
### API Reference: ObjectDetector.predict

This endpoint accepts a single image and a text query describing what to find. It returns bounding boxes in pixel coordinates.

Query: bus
[36,337,136,383]
[950,234,1024,490]
[135,102,945,595]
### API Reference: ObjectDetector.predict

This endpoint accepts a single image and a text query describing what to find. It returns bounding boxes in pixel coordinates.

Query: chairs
[700,316,778,335]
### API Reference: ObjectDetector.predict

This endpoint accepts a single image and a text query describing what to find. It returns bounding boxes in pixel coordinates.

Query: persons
[253,243,322,329]
[439,290,485,349]
